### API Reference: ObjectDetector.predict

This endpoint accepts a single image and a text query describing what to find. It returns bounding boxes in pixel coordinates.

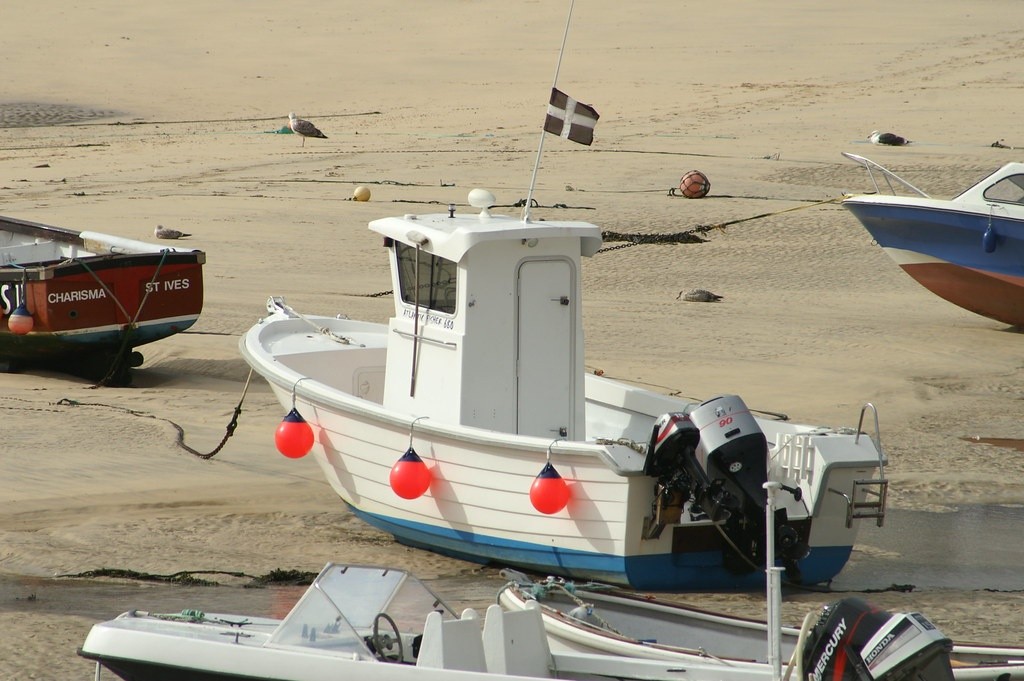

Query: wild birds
[154,223,193,240]
[866,129,915,147]
[675,288,724,303]
[288,111,329,148]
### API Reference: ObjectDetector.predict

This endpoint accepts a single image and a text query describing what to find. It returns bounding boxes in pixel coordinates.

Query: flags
[544,87,598,145]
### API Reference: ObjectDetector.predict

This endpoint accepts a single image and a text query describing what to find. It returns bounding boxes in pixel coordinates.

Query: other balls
[680,170,710,199]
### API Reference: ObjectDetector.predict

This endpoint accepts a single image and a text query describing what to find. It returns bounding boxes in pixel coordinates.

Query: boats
[75,564,556,681]
[0,215,207,367]
[839,151,1024,328]
[238,187,890,591]
[496,562,1024,681]
